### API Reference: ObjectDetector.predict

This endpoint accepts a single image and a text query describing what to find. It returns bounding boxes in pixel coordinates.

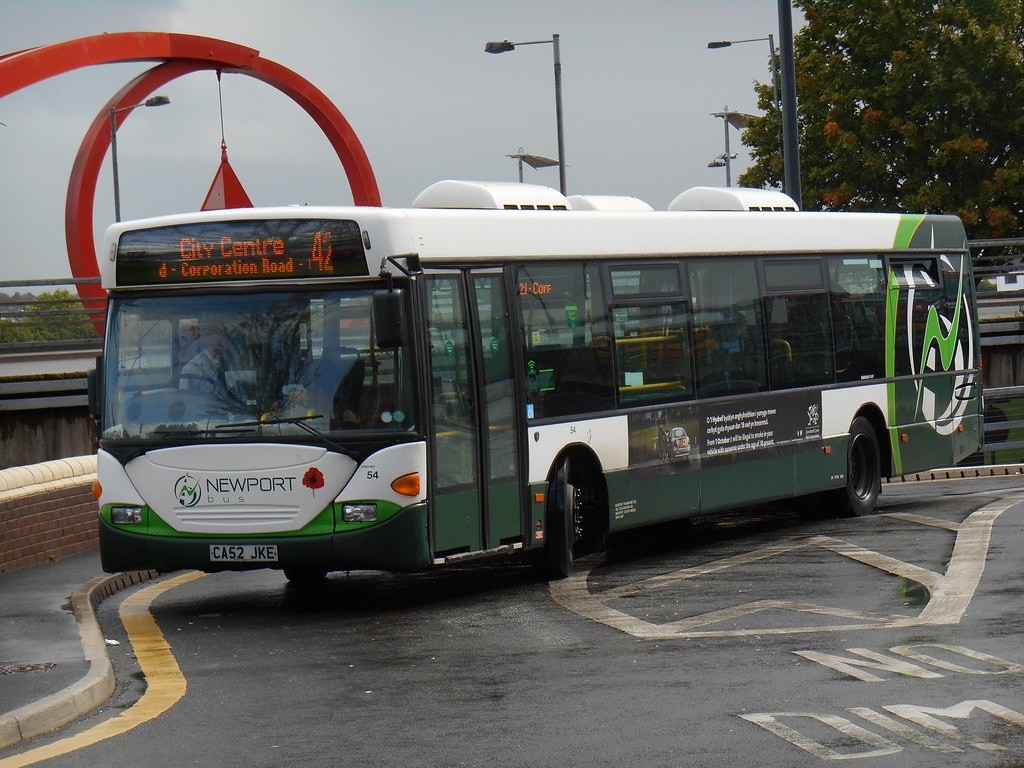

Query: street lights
[112,95,173,222]
[705,33,786,193]
[483,31,567,198]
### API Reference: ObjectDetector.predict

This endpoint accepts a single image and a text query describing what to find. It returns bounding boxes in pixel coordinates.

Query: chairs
[313,289,929,427]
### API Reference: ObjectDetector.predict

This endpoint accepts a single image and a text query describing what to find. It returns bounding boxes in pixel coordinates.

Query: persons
[178,334,249,404]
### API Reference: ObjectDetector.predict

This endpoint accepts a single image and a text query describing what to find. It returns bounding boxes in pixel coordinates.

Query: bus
[87,177,987,598]
